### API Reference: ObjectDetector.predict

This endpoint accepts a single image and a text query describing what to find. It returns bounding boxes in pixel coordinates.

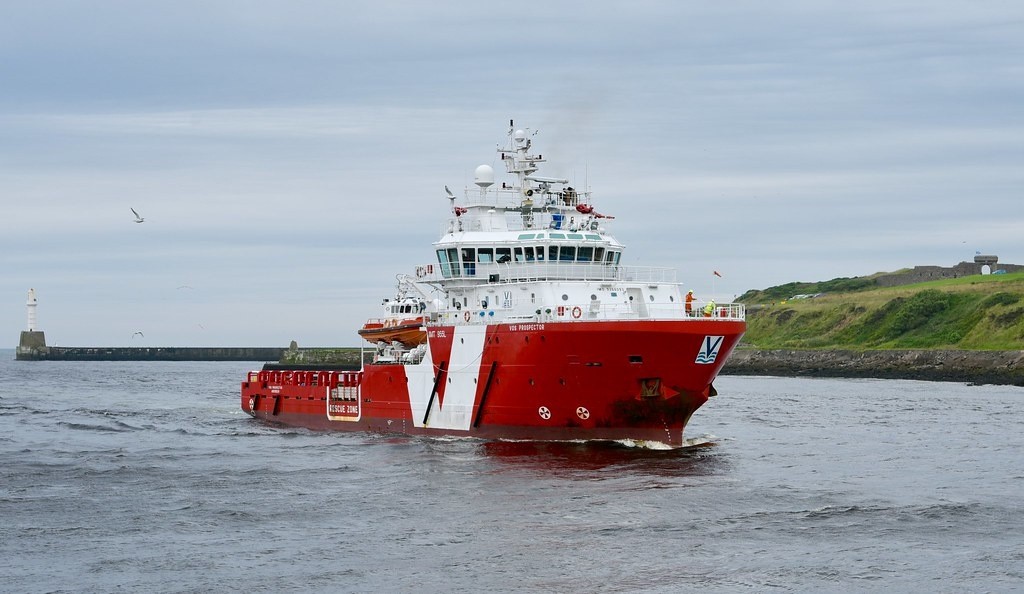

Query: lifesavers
[464,311,471,322]
[571,306,582,319]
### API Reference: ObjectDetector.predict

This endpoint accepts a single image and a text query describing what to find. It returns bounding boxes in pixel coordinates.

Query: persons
[703,299,717,317]
[685,290,697,317]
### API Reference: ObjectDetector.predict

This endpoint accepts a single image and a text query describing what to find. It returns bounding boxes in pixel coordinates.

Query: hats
[689,290,693,292]
[711,299,714,301]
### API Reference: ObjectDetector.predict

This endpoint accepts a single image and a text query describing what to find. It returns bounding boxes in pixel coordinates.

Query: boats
[242,119,747,453]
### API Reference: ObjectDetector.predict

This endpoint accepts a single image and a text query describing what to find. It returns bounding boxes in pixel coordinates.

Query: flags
[714,271,721,277]
